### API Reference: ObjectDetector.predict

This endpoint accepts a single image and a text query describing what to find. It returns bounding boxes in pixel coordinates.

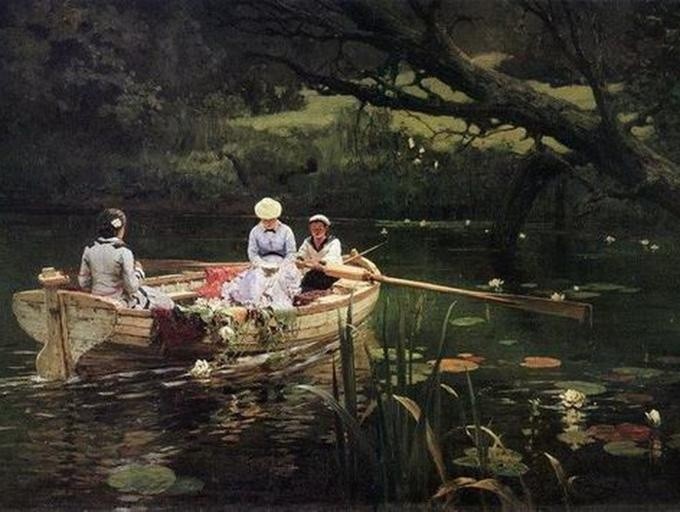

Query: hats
[308,214,331,227]
[254,196,282,219]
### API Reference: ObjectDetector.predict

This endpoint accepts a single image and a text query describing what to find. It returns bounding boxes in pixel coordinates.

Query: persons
[227,198,302,307]
[295,212,344,292]
[77,207,183,311]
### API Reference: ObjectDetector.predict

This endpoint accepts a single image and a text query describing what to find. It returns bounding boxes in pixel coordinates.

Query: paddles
[296,259,592,322]
[138,257,251,274]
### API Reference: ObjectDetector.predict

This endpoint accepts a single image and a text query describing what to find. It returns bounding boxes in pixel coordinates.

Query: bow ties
[265,228,275,233]
